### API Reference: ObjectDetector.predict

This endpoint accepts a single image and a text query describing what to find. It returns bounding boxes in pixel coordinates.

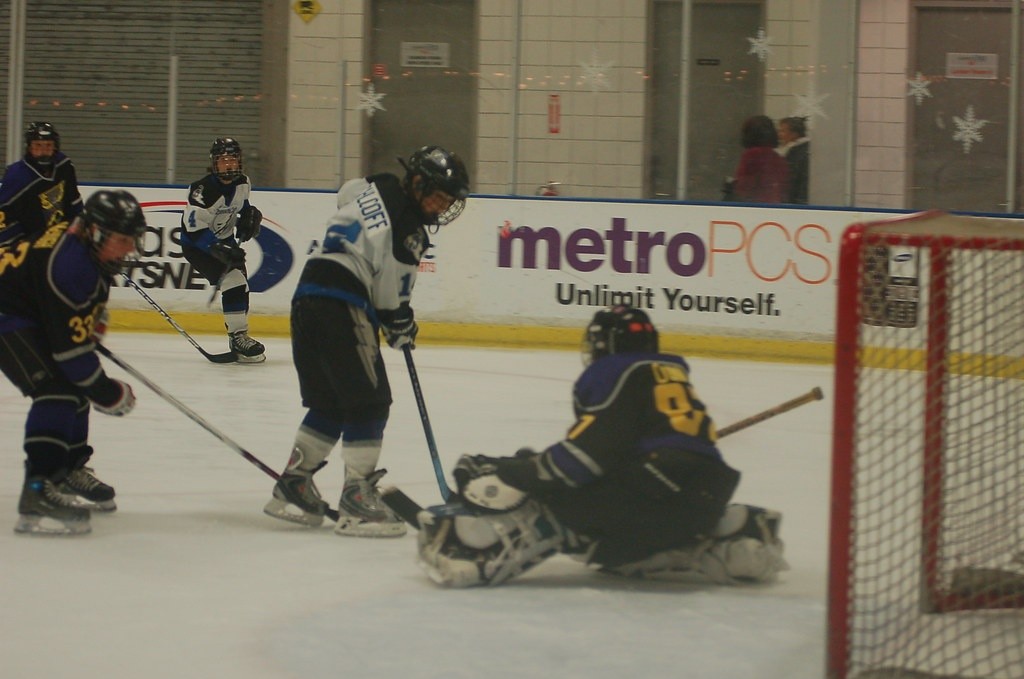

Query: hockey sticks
[202,236,243,316]
[120,270,238,364]
[402,345,457,504]
[380,385,827,531]
[96,341,340,523]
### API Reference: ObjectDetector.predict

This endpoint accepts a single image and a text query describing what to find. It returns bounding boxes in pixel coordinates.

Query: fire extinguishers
[536,180,560,196]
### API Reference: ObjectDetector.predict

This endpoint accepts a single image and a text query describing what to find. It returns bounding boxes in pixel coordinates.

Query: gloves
[91,378,135,415]
[381,300,418,351]
[235,205,263,242]
[496,450,576,503]
[212,241,246,266]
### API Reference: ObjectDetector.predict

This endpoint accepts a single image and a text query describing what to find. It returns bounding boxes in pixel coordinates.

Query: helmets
[405,143,469,226]
[26,120,61,169]
[210,137,243,181]
[82,188,147,272]
[580,303,658,368]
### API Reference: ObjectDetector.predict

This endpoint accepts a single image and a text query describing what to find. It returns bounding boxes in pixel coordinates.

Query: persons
[181,136,266,363]
[0,190,149,538]
[263,145,469,537]
[722,115,790,202]
[0,120,82,254]
[416,303,783,589]
[778,117,808,205]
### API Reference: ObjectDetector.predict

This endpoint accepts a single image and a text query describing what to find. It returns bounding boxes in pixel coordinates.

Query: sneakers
[14,459,92,534]
[261,448,327,525]
[45,445,117,511]
[335,465,407,537]
[225,321,266,362]
